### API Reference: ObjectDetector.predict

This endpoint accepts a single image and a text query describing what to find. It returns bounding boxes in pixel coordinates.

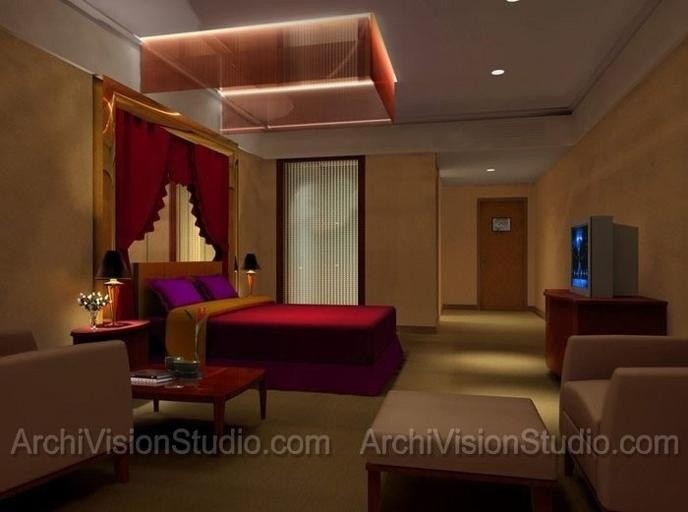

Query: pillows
[144,275,204,312]
[191,274,239,301]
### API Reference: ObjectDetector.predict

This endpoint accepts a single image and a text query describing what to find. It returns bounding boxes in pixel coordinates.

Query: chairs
[1,328,134,511]
[559,334,688,512]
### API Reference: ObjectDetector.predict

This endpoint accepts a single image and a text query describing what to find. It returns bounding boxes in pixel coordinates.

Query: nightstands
[70,319,150,371]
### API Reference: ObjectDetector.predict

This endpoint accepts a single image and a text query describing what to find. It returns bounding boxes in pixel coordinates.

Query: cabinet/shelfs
[544,288,668,380]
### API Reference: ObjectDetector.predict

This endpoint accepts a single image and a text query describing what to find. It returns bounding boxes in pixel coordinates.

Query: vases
[88,309,98,328]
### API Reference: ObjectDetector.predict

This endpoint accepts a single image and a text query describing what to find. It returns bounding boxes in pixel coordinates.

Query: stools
[363,389,567,511]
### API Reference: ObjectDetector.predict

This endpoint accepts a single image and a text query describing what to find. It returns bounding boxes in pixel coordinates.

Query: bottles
[94,310,103,328]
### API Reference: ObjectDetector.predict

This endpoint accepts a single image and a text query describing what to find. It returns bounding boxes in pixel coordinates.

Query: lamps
[241,254,262,296]
[93,251,133,327]
[140,13,397,135]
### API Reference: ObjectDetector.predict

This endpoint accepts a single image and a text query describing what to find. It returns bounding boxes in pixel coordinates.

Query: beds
[136,261,403,397]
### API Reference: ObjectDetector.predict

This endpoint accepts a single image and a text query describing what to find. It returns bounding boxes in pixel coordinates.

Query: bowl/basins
[172,360,200,375]
[174,372,203,383]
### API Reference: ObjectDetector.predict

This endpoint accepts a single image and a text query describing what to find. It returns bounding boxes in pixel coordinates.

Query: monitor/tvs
[570,214,644,298]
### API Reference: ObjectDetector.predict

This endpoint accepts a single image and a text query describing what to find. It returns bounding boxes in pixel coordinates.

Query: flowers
[76,289,109,310]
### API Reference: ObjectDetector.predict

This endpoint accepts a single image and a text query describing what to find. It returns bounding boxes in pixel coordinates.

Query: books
[130,376,174,387]
[135,369,171,379]
